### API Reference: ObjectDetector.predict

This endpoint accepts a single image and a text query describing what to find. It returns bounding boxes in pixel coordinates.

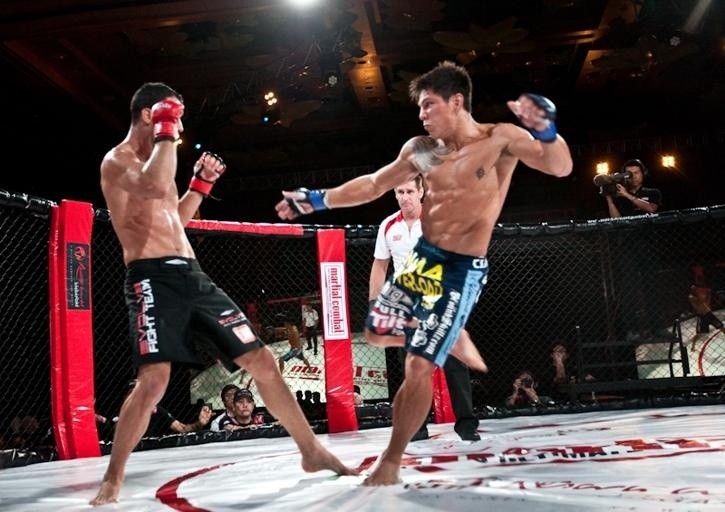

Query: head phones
[623,159,651,187]
[510,371,539,391]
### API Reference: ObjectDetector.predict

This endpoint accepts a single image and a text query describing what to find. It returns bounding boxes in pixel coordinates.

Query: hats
[234,389,252,401]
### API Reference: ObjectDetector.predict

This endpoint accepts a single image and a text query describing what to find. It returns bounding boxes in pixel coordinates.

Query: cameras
[521,378,532,388]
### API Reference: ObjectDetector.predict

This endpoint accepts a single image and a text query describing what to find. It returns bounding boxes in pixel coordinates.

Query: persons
[266,316,311,375]
[274,59,574,487]
[598,159,659,218]
[687,284,725,351]
[505,339,594,407]
[0,384,264,449]
[367,176,480,441]
[87,81,361,506]
[302,302,319,355]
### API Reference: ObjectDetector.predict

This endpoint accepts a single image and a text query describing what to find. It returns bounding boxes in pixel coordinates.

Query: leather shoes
[411,429,428,441]
[454,423,480,440]
